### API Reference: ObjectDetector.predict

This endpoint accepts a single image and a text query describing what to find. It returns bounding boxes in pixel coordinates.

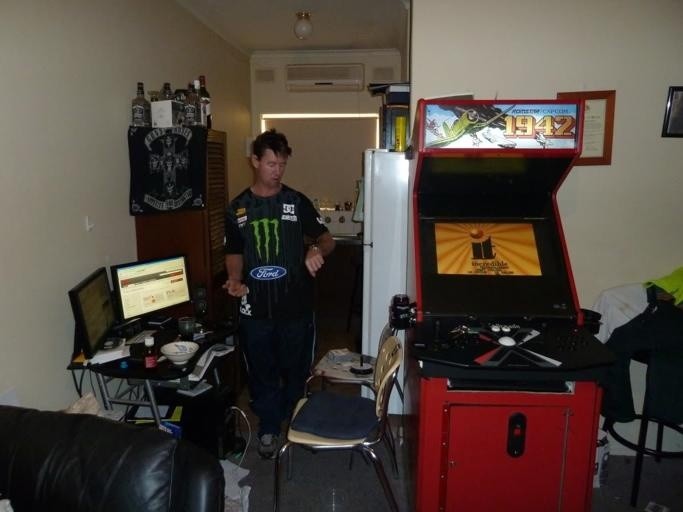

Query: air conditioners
[284,62,366,93]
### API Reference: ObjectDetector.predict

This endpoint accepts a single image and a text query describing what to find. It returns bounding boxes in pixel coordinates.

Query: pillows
[0,405,179,512]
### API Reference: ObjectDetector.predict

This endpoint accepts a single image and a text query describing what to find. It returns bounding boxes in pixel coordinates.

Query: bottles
[141,336,158,372]
[131,73,211,129]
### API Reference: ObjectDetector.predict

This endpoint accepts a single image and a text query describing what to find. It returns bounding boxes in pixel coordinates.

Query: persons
[221,129,337,460]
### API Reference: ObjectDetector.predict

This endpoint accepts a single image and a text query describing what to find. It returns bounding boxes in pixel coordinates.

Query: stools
[346,254,362,335]
[601,350,683,507]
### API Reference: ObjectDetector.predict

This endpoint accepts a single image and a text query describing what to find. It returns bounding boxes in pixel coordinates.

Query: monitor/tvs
[421,218,573,311]
[68,266,116,359]
[111,255,193,321]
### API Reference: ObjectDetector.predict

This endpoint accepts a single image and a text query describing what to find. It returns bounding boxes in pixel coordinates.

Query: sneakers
[257,434,279,459]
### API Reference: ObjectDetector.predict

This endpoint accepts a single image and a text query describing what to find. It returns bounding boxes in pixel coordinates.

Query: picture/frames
[555,90,616,167]
[662,86,683,137]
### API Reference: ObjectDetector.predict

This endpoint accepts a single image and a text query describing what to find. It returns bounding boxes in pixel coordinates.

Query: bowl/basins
[160,342,199,365]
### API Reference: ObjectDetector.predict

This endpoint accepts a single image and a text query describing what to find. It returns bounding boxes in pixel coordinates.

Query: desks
[66,319,240,427]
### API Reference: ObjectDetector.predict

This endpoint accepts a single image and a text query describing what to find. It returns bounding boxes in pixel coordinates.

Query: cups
[312,198,352,210]
[178,316,195,342]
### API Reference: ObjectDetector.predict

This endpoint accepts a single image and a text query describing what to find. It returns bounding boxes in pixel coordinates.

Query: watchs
[309,244,320,250]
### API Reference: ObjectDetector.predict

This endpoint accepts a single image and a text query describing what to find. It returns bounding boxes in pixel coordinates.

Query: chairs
[173,439,225,512]
[305,322,404,472]
[273,336,404,512]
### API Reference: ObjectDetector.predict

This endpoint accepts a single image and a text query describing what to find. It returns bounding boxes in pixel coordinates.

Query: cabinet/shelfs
[133,128,247,404]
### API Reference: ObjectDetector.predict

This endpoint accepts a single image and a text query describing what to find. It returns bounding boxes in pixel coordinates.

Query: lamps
[293,7,315,41]
